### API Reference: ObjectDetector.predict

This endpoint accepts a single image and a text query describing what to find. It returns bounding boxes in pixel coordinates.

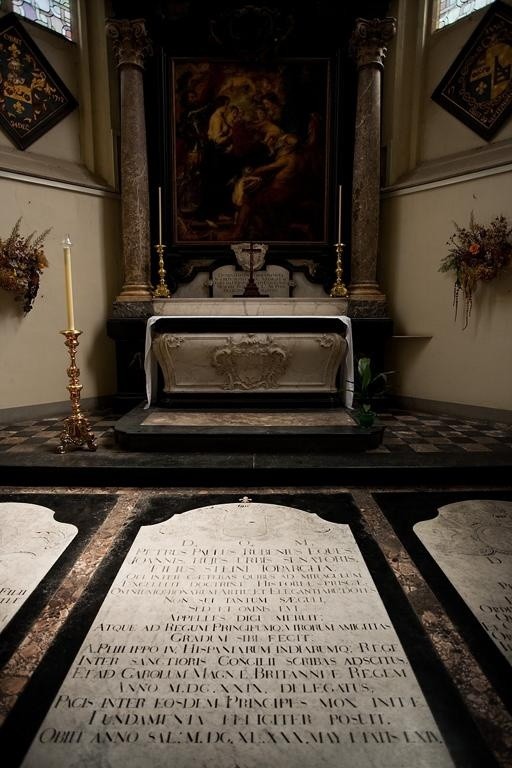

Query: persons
[180,90,298,236]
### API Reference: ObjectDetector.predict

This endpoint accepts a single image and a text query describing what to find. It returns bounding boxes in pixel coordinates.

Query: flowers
[0,213,55,317]
[438,208,511,328]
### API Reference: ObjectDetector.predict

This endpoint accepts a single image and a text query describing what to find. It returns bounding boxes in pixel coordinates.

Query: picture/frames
[0,10,80,150]
[157,46,340,254]
[429,0,512,144]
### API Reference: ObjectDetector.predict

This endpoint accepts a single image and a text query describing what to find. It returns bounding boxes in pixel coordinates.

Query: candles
[156,186,163,246]
[62,246,76,330]
[337,184,343,244]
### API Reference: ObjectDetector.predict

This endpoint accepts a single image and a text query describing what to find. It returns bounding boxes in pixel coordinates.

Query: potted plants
[345,357,396,452]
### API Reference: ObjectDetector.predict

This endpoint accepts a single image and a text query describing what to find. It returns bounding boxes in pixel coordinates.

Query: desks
[151,317,346,410]
